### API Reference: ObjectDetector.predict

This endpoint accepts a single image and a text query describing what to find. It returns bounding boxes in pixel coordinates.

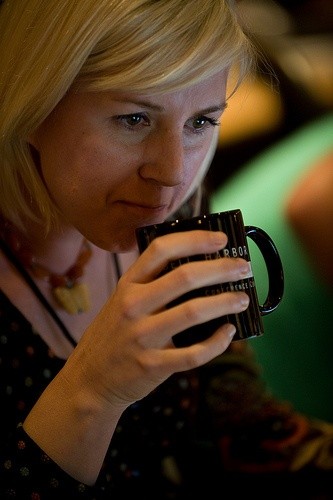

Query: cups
[135,206,286,359]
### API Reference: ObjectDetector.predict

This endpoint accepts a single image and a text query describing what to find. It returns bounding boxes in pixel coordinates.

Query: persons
[0,0,333,500]
[286,143,333,282]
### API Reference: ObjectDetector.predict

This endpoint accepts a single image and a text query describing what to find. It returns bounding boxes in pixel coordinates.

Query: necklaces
[0,239,120,354]
[0,224,92,315]
